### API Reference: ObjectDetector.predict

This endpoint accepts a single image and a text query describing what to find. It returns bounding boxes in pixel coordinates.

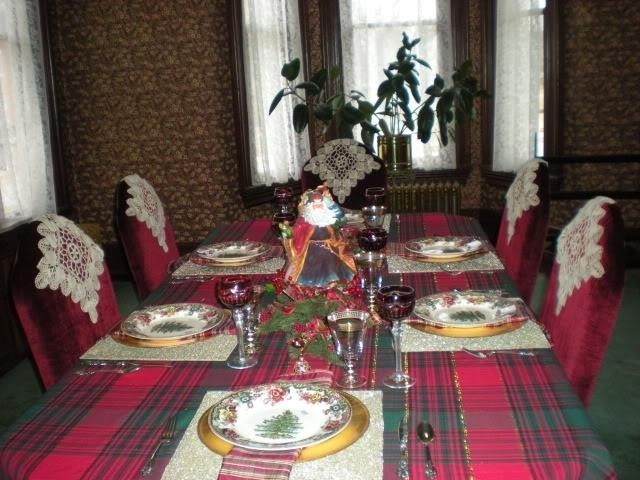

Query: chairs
[535,196,625,405]
[1,214,123,392]
[491,157,550,302]
[110,173,180,300]
[299,140,389,212]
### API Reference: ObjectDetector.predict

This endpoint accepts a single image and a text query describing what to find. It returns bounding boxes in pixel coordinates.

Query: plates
[197,379,369,462]
[407,290,533,338]
[112,302,231,348]
[401,233,490,263]
[188,240,277,266]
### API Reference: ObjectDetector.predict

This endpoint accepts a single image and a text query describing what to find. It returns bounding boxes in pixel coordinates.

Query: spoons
[416,420,442,480]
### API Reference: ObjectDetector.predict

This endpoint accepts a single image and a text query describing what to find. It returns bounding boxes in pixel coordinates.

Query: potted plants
[268,32,489,170]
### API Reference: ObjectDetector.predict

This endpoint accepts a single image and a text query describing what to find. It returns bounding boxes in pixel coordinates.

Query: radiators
[380,178,462,214]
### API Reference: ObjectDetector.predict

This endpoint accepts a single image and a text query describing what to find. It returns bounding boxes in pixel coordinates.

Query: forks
[437,263,496,278]
[460,347,540,361]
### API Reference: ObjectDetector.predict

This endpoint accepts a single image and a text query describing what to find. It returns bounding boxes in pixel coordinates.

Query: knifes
[398,410,410,479]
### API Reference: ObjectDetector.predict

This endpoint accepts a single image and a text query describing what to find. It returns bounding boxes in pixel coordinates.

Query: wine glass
[326,308,370,388]
[378,285,416,391]
[354,229,390,324]
[216,267,267,372]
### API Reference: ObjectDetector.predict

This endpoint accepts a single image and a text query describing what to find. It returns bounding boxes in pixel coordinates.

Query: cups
[362,186,385,228]
[271,187,295,237]
